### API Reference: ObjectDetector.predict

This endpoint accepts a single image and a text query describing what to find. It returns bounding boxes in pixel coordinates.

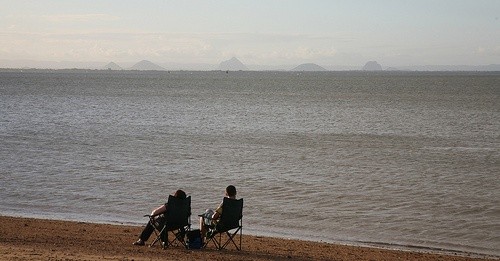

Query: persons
[200,185,236,248]
[132,189,188,247]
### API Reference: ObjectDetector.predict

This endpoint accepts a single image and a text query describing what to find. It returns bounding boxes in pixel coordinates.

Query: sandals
[131,240,145,245]
[162,242,167,245]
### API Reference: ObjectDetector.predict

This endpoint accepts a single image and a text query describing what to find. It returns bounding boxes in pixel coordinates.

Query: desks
[187,229,202,250]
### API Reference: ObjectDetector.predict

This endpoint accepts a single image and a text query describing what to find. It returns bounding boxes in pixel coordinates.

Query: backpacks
[189,229,203,248]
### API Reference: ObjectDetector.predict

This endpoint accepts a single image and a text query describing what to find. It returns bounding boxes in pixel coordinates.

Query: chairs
[144,196,192,251]
[197,197,244,252]
